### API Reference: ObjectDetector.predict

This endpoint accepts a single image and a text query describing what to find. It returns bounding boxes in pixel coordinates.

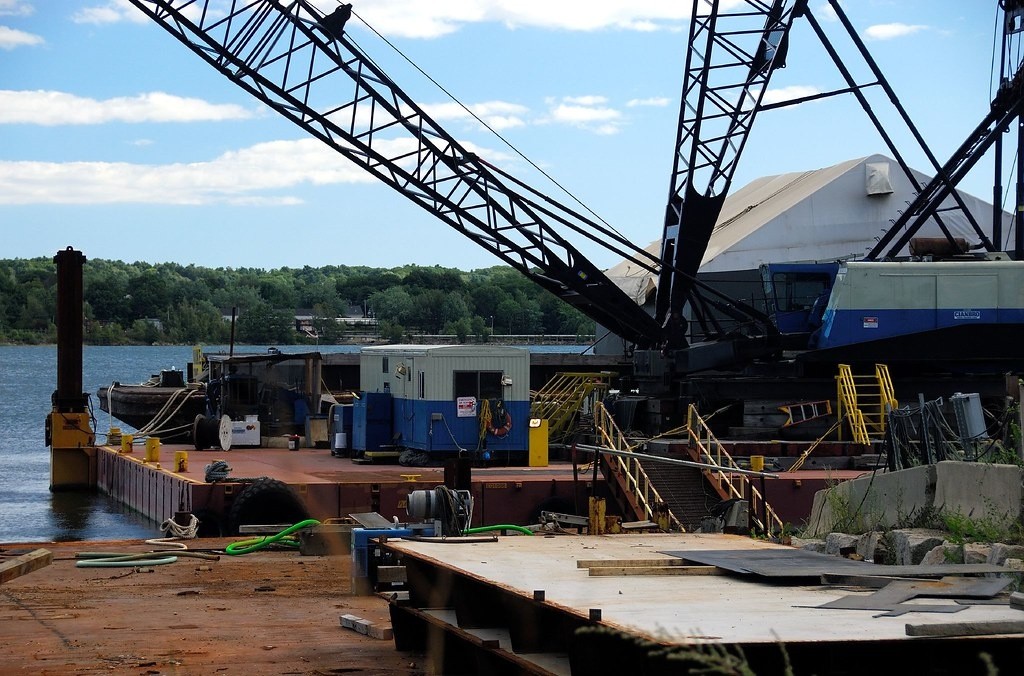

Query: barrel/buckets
[751,456,763,471]
[335,433,347,449]
[288,436,300,451]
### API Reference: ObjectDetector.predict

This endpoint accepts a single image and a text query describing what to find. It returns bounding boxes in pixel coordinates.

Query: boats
[98,307,361,443]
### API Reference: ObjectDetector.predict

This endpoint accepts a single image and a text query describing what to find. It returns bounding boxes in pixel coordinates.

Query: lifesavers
[487,412,512,436]
[229,477,311,536]
